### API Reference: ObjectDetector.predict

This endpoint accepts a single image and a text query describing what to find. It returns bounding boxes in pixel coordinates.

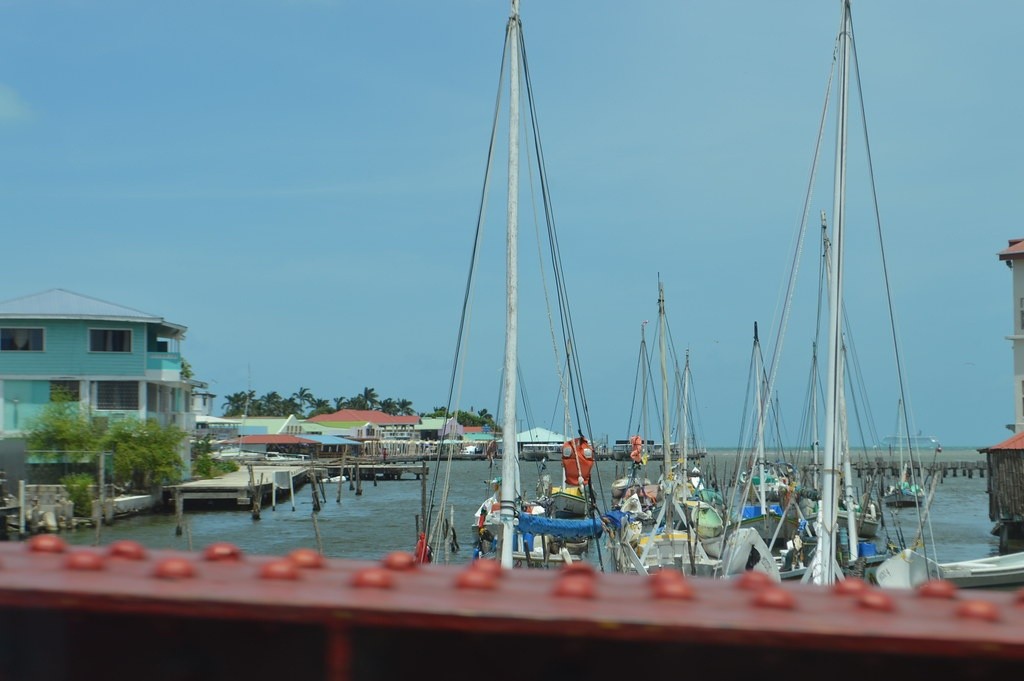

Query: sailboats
[413,0,1024,594]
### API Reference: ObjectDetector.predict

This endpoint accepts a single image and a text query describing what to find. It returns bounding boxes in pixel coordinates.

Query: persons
[382,448,389,461]
